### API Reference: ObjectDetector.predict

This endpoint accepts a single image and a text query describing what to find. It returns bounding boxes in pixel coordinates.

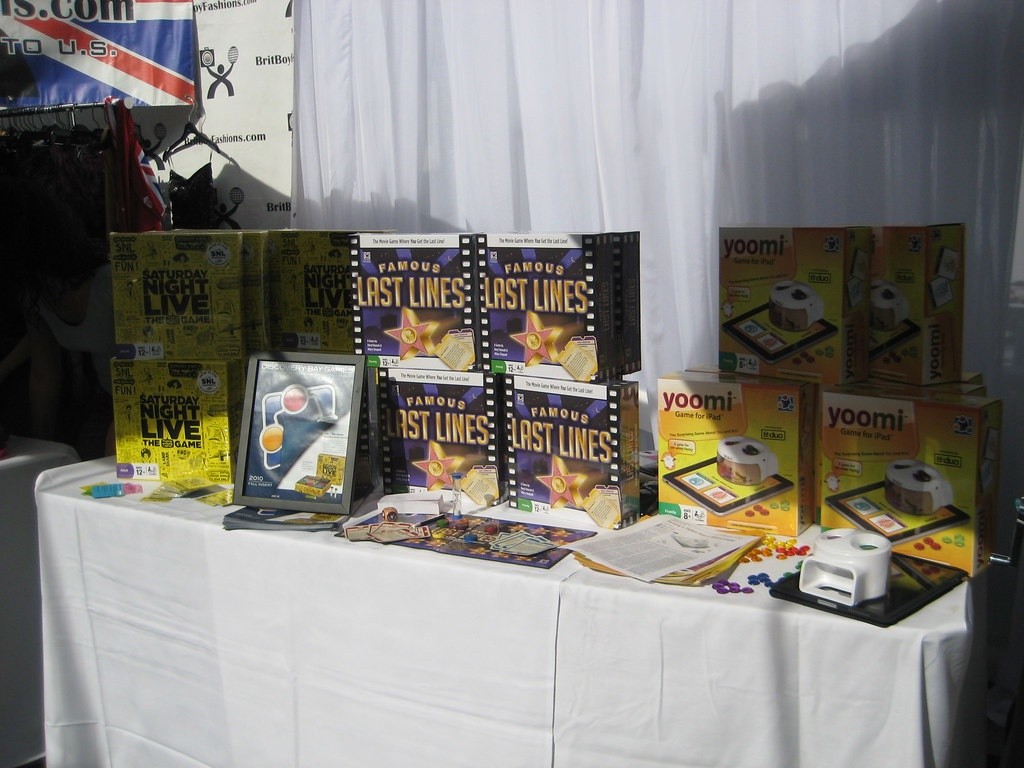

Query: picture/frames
[232,350,365,516]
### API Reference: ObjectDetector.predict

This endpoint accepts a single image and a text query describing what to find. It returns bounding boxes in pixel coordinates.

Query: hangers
[0,102,114,168]
[162,96,219,162]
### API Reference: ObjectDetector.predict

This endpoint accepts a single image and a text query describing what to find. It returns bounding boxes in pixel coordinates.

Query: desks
[33,453,987,768]
[0,436,82,768]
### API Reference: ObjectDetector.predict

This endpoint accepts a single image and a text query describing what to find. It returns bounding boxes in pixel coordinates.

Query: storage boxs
[349,232,484,371]
[270,228,397,355]
[821,388,1003,576]
[109,232,244,361]
[718,225,871,386]
[655,375,814,532]
[870,223,965,387]
[504,377,635,530]
[147,231,271,353]
[477,231,615,377]
[606,228,641,377]
[111,360,241,486]
[375,367,506,506]
